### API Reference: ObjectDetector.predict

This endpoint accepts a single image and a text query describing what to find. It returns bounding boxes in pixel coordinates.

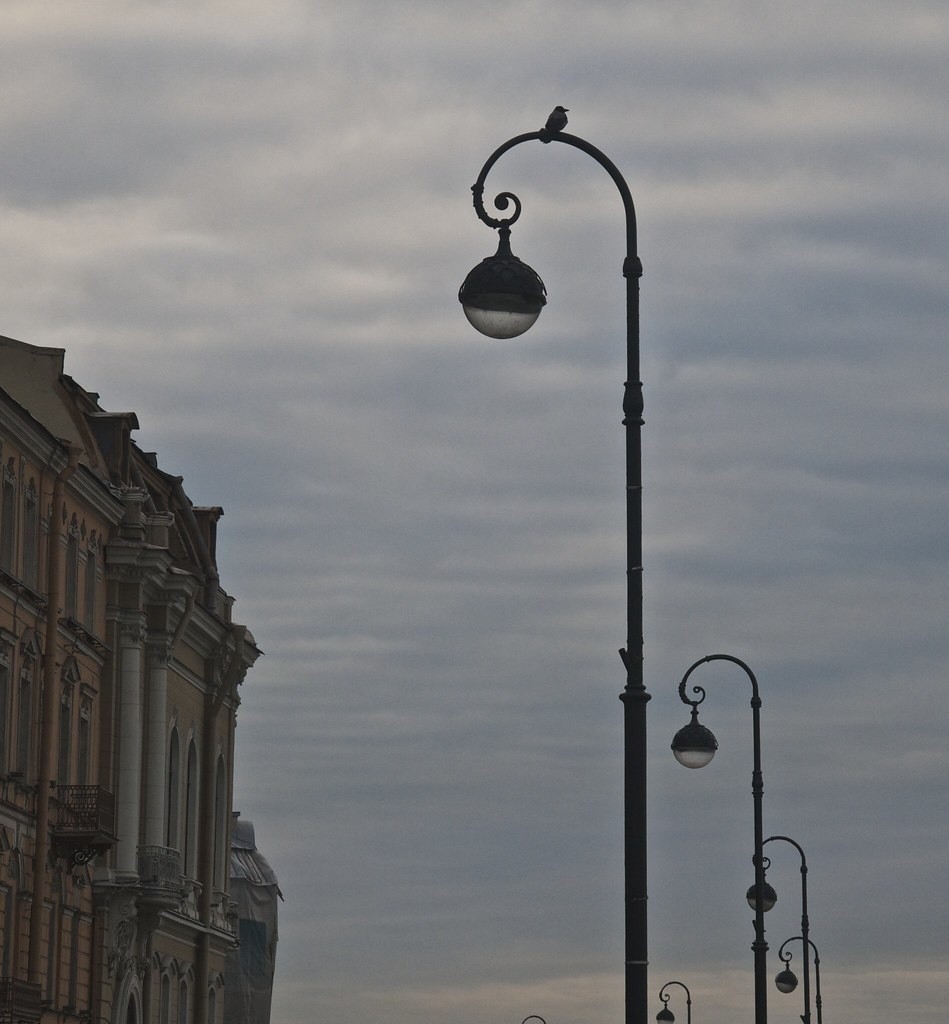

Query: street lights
[742,834,812,1024]
[655,981,693,1024]
[458,123,648,1024]
[666,652,772,1024]
[774,936,824,1024]
